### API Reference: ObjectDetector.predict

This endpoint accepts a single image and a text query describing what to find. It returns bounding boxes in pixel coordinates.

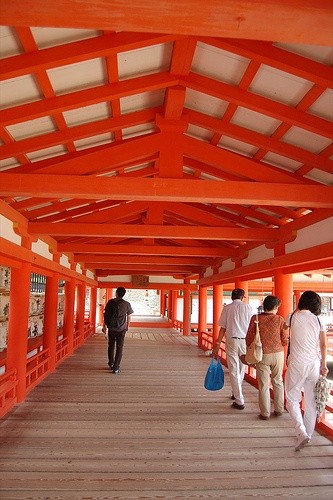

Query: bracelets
[215,341,220,345]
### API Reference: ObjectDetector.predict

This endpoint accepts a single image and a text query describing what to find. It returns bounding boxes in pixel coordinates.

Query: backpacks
[104,299,124,328]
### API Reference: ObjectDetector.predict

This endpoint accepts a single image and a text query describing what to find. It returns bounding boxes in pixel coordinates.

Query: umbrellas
[313,369,331,423]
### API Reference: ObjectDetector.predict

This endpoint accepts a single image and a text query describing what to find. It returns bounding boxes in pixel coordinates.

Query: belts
[231,337,246,340]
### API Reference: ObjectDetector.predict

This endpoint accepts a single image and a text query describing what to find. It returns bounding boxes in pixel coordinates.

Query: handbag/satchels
[246,315,263,365]
[204,351,224,391]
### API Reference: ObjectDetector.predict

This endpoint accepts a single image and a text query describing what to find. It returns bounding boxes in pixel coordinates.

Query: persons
[212,288,255,410]
[101,287,134,373]
[245,295,289,420]
[282,291,328,450]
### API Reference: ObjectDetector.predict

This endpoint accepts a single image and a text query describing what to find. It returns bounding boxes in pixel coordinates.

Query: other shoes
[259,413,269,420]
[108,363,113,368]
[233,402,245,409]
[294,432,310,451]
[274,410,282,416]
[113,368,121,374]
